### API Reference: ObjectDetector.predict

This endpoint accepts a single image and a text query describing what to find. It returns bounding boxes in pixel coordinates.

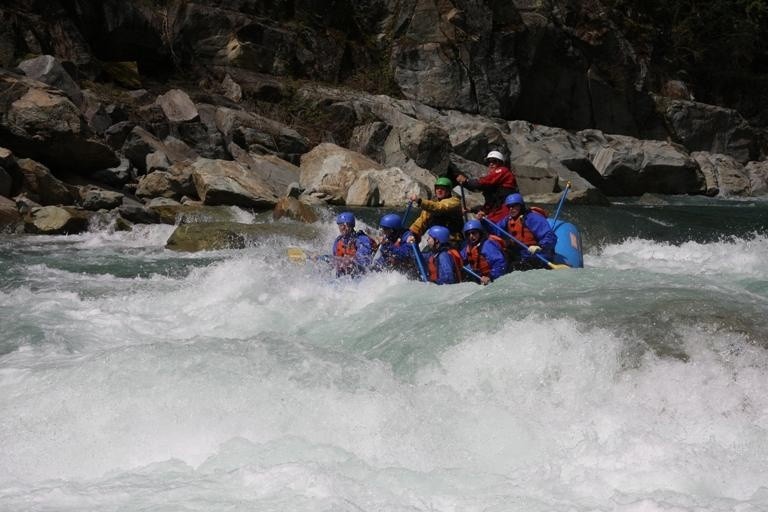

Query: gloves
[528,245,542,255]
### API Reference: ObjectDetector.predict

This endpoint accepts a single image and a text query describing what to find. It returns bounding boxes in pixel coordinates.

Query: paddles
[452,185,484,205]
[482,216,571,269]
[287,249,332,262]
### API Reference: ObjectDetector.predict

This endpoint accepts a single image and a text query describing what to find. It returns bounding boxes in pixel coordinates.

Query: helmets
[379,214,401,232]
[464,220,482,238]
[336,212,355,227]
[428,225,449,244]
[505,193,525,210]
[435,178,453,192]
[486,151,505,166]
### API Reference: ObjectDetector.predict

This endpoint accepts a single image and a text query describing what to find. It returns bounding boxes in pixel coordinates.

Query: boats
[546,214,584,269]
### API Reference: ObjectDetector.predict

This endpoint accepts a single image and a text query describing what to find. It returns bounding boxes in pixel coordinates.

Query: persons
[407,225,464,285]
[310,212,377,277]
[372,213,417,278]
[456,150,519,223]
[476,192,558,271]
[408,177,465,250]
[460,219,509,286]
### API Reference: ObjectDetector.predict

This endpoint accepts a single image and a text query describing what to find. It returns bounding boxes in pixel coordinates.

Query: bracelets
[415,196,423,207]
